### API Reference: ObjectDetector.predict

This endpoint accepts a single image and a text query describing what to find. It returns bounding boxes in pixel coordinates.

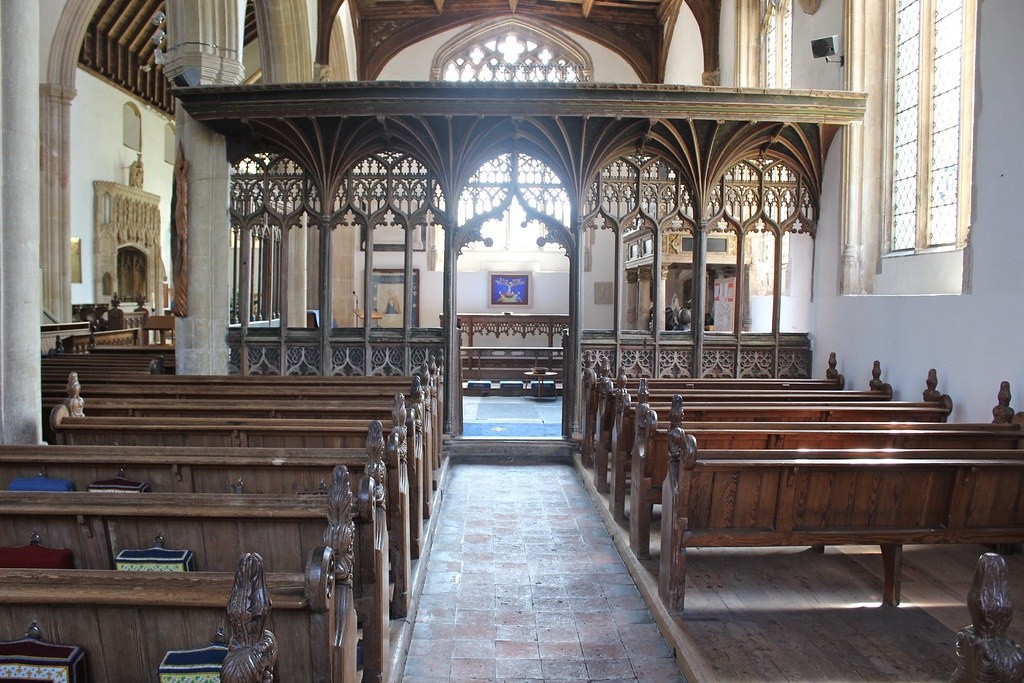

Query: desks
[524,371,557,401]
[439,313,571,381]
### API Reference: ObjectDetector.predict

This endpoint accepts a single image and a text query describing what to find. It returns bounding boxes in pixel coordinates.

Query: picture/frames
[488,271,532,309]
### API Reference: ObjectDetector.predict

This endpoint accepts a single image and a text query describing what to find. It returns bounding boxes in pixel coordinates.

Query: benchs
[582,350,1024,618]
[0,293,444,683]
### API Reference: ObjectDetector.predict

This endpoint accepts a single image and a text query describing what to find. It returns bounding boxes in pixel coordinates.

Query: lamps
[151,30,168,45]
[154,49,168,65]
[152,12,166,25]
